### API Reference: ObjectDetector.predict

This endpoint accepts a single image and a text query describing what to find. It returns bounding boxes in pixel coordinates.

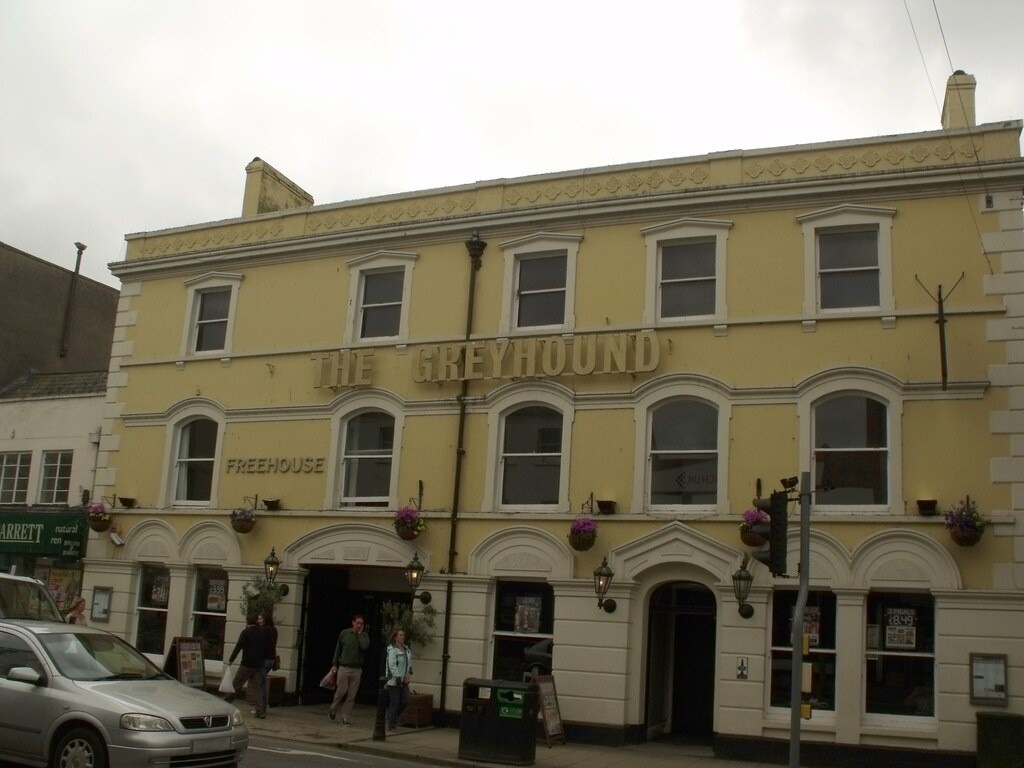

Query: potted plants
[238,576,286,706]
[380,600,437,728]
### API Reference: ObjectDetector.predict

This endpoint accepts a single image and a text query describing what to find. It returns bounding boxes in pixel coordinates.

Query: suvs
[0,572,66,623]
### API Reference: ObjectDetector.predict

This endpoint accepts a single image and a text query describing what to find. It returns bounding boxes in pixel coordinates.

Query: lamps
[265,546,288,597]
[596,500,615,515]
[733,561,753,619]
[119,498,135,509]
[405,551,432,603]
[917,499,936,515]
[594,556,616,613]
[262,499,281,510]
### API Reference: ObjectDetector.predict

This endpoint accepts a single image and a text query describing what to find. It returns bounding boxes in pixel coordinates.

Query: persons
[386,629,413,732]
[329,611,370,726]
[226,611,266,719]
[64,596,96,659]
[251,610,278,712]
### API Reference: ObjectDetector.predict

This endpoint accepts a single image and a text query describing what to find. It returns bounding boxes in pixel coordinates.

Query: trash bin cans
[457,677,540,766]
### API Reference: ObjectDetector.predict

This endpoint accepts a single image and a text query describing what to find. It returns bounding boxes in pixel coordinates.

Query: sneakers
[338,718,351,727]
[329,708,336,720]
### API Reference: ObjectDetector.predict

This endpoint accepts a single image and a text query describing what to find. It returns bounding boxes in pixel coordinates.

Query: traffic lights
[751,489,788,578]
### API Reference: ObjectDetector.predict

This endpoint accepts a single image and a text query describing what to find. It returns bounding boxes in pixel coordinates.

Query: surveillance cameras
[111,533,125,546]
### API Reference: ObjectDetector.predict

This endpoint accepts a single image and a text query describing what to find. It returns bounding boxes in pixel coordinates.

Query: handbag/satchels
[272,655,280,671]
[218,665,235,693]
[320,670,336,690]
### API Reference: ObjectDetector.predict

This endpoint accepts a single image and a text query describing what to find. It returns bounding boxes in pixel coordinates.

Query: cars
[0,618,249,768]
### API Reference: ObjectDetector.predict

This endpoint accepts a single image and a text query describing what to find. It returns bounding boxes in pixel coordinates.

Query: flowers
[570,517,598,534]
[944,495,992,530]
[230,506,256,522]
[738,509,771,531]
[84,503,112,521]
[392,505,427,535]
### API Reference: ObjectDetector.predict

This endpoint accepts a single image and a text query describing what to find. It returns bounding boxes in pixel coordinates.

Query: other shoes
[254,712,266,720]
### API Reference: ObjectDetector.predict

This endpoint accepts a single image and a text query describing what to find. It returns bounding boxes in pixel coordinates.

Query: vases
[231,520,257,532]
[395,526,422,541]
[741,531,769,546]
[950,528,983,546]
[569,533,596,551]
[89,519,111,531]
